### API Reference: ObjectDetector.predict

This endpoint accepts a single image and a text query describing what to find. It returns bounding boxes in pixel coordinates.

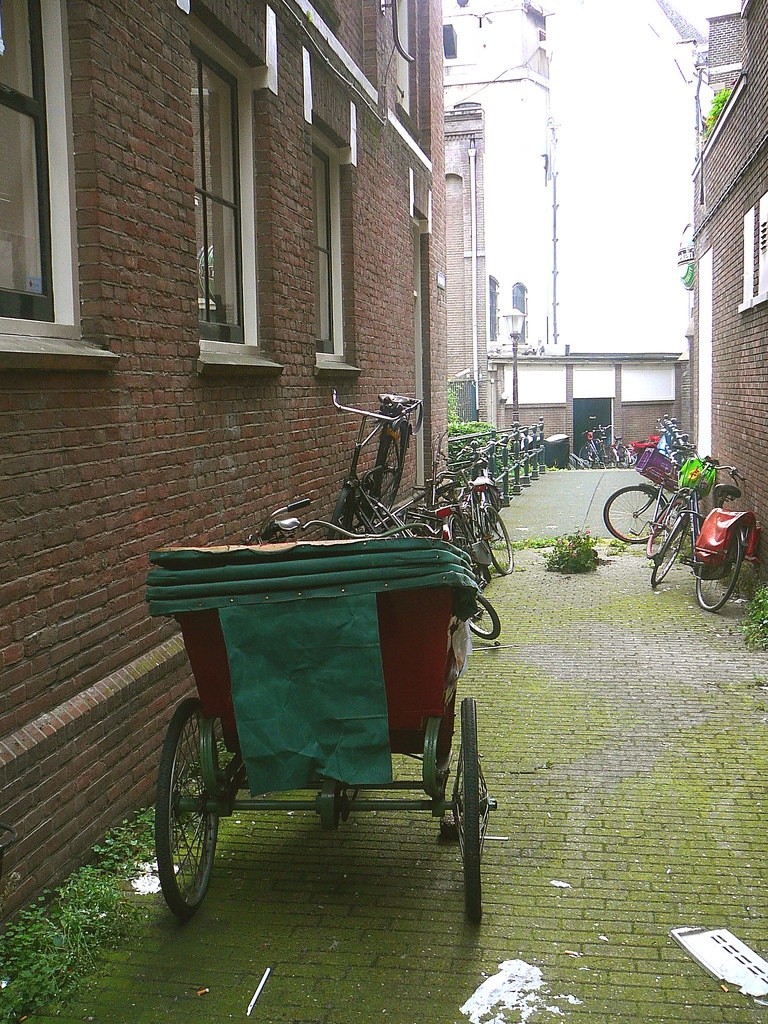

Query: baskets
[635,447,682,492]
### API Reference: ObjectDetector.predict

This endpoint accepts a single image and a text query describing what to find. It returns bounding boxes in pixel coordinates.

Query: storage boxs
[635,447,679,489]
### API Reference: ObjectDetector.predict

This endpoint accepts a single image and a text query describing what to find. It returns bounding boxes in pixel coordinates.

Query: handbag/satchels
[695,507,760,567]
[677,457,716,497]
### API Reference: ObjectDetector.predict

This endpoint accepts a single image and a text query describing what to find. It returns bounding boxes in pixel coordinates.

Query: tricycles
[145,519,496,922]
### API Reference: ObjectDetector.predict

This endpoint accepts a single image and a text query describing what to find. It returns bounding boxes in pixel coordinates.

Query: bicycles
[355,439,513,639]
[604,442,689,558]
[649,445,755,613]
[579,429,619,470]
[594,425,638,469]
[329,387,423,539]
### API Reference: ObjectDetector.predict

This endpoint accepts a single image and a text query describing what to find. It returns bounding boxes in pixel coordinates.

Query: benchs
[146,538,484,736]
